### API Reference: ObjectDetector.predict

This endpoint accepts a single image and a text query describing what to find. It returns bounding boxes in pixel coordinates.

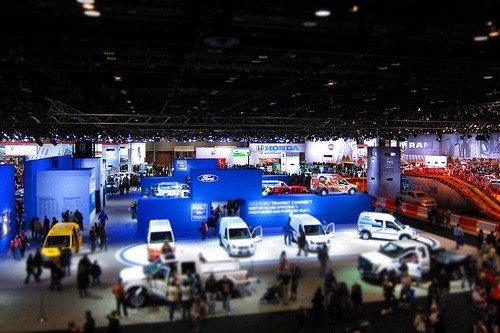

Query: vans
[219,216,263,257]
[358,212,418,240]
[309,174,358,196]
[146,220,177,260]
[42,221,82,263]
[152,182,190,197]
[290,214,335,252]
[261,180,308,194]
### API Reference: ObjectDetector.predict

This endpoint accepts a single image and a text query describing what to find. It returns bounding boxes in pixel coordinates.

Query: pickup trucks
[119,252,250,309]
[396,190,438,209]
[357,240,473,286]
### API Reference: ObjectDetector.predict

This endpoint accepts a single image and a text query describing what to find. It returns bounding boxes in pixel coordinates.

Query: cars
[107,174,120,191]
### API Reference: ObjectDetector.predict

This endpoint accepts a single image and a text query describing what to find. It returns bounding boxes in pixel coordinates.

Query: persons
[10,209,108,261]
[199,221,208,240]
[454,224,464,249]
[427,206,454,230]
[0,230,500,333]
[319,245,330,277]
[212,200,240,216]
[282,222,295,245]
[231,161,367,191]
[129,201,137,219]
[98,210,108,227]
[119,162,172,197]
